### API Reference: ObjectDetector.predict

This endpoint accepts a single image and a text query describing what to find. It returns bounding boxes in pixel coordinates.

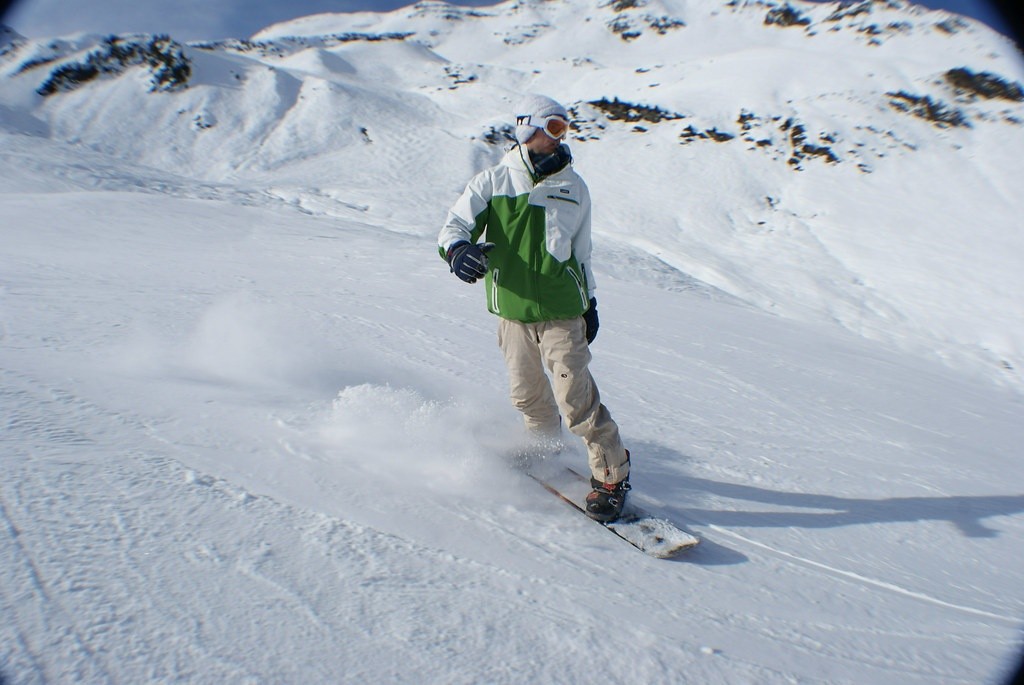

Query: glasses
[520,114,568,139]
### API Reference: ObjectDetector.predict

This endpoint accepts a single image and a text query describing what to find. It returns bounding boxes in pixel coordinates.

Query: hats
[515,94,569,144]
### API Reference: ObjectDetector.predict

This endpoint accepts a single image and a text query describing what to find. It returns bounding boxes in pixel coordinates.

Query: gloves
[583,297,599,345]
[445,240,495,284]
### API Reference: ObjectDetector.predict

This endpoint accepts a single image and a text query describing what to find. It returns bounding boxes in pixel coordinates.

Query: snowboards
[465,411,700,559]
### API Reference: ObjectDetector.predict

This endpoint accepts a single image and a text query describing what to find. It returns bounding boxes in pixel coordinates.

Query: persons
[437,93,631,522]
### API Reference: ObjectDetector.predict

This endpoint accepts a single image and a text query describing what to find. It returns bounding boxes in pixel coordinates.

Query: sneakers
[587,472,632,524]
